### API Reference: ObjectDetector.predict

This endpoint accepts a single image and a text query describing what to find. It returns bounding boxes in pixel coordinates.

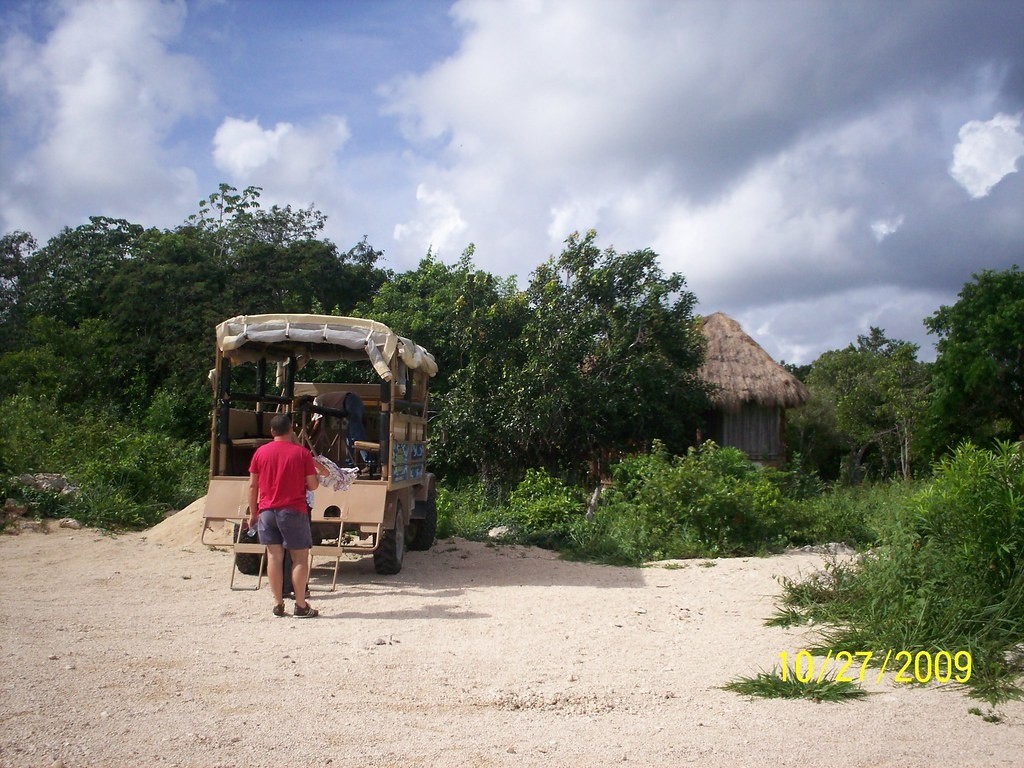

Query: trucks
[202,313,438,576]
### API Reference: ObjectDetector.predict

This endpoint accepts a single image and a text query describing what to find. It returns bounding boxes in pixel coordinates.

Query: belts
[343,393,350,411]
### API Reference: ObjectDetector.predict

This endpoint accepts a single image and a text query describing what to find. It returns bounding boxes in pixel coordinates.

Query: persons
[296,391,378,474]
[247,414,330,618]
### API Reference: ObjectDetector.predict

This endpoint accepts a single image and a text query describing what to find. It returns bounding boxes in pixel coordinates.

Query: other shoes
[361,465,376,473]
[282,593,291,598]
[294,590,310,598]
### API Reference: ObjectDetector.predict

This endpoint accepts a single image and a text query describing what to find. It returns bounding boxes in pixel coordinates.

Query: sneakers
[273,604,283,617]
[293,602,318,618]
